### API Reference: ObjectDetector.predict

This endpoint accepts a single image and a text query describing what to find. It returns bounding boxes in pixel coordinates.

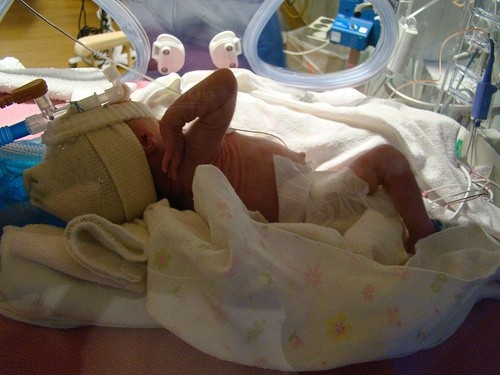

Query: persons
[24,68,463,255]
